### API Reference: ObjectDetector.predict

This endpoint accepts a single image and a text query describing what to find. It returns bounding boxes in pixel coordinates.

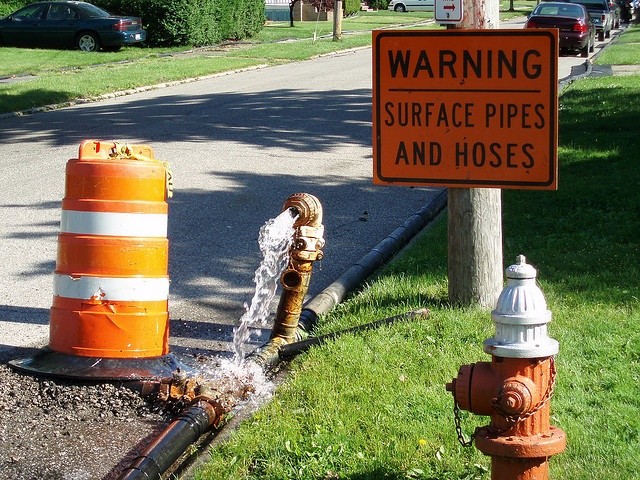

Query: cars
[523,2,596,57]
[388,0,434,12]
[0,1,147,52]
[608,1,621,29]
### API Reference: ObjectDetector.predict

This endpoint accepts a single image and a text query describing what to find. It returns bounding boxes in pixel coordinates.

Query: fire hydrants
[445,254,566,479]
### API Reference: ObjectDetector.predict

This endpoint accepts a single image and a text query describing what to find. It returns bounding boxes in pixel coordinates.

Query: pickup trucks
[569,0,613,41]
